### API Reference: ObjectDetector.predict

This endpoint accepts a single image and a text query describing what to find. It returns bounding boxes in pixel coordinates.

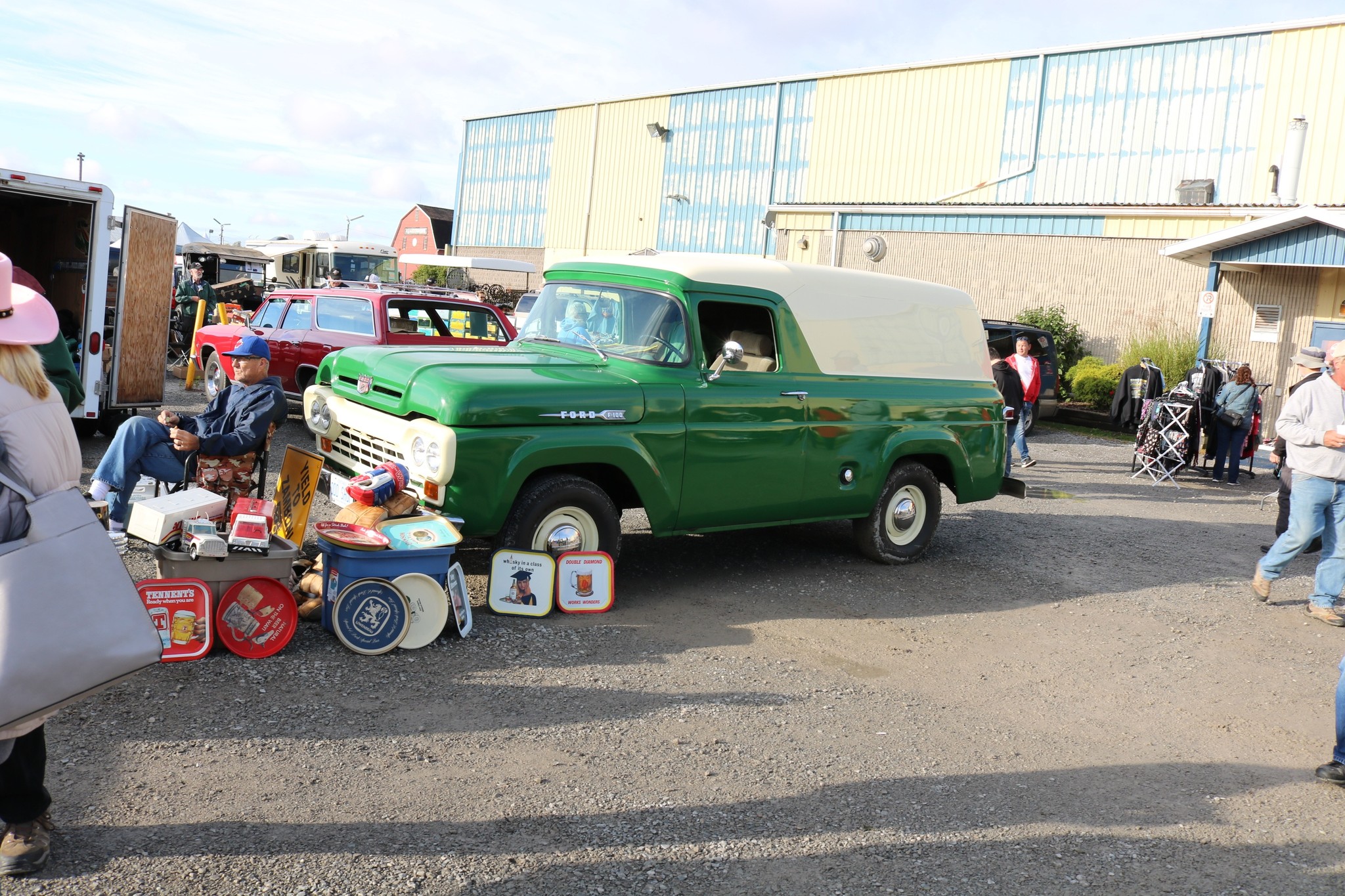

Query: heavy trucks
[165,511,228,562]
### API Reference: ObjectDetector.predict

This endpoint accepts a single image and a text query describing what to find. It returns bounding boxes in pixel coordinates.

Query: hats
[190,262,205,270]
[1331,339,1345,359]
[1289,346,1329,368]
[0,252,59,345]
[364,273,380,282]
[329,267,342,279]
[221,336,271,361]
[425,277,437,285]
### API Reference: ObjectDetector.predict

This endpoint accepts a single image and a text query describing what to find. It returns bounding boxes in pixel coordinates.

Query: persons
[175,263,382,367]
[1259,345,1326,555]
[475,291,485,301]
[1004,337,1041,469]
[0,249,81,870]
[1314,655,1345,783]
[1213,366,1256,486]
[988,347,1025,478]
[88,335,288,531]
[454,287,462,290]
[558,302,591,345]
[399,272,402,291]
[424,278,443,295]
[1254,340,1345,626]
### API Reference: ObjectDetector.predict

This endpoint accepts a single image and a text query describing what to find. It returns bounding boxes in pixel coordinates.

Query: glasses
[479,296,485,299]
[366,284,372,287]
[194,270,204,273]
[1016,337,1029,344]
[578,312,587,316]
[231,356,262,363]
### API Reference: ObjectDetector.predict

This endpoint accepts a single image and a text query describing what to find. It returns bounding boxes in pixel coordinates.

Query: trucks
[244,237,400,303]
[228,497,277,556]
[172,242,277,343]
[0,169,178,439]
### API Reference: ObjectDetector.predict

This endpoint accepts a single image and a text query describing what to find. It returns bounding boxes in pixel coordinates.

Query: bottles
[510,579,517,600]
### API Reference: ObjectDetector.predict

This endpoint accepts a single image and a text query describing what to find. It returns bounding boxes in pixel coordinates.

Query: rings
[181,441,183,445]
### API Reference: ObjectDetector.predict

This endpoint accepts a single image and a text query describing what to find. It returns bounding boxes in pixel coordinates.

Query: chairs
[709,331,776,372]
[166,310,191,372]
[154,421,276,530]
[640,322,671,362]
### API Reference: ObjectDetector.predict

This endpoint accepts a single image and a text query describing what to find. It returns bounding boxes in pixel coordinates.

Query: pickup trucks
[302,253,1026,568]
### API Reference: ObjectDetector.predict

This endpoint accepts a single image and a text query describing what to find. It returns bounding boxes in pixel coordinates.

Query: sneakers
[1303,600,1345,627]
[1010,461,1015,466]
[1252,561,1271,602]
[1021,459,1036,468]
[0,807,56,874]
[1313,759,1345,784]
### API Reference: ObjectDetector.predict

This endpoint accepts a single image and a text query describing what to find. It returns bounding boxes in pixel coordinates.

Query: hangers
[1164,380,1200,400]
[1188,358,1242,376]
[1124,357,1161,378]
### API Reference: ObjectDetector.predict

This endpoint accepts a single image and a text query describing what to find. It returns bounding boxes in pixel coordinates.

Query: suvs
[513,290,606,340]
[981,319,1059,440]
[189,281,521,440]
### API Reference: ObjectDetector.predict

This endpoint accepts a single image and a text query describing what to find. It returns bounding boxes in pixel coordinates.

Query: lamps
[862,235,887,262]
[797,235,807,249]
[646,122,670,137]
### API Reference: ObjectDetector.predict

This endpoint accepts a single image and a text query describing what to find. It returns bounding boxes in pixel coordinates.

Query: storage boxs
[212,303,255,324]
[146,532,299,649]
[173,366,204,379]
[105,479,155,529]
[316,536,455,633]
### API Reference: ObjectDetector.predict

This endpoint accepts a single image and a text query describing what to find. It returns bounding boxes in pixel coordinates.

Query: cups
[171,613,200,645]
[1337,424,1345,445]
[506,593,512,601]
[570,569,594,597]
[516,593,521,600]
[221,601,260,642]
[237,584,264,611]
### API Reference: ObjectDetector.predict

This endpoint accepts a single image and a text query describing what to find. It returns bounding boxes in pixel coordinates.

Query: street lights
[346,215,365,241]
[213,219,231,245]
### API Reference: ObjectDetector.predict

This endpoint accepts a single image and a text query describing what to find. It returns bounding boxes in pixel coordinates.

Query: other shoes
[1212,478,1224,482]
[1260,544,1274,553]
[1227,480,1241,485]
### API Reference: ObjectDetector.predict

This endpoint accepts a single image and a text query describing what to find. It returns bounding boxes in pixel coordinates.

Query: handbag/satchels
[1218,410,1243,429]
[0,458,165,727]
